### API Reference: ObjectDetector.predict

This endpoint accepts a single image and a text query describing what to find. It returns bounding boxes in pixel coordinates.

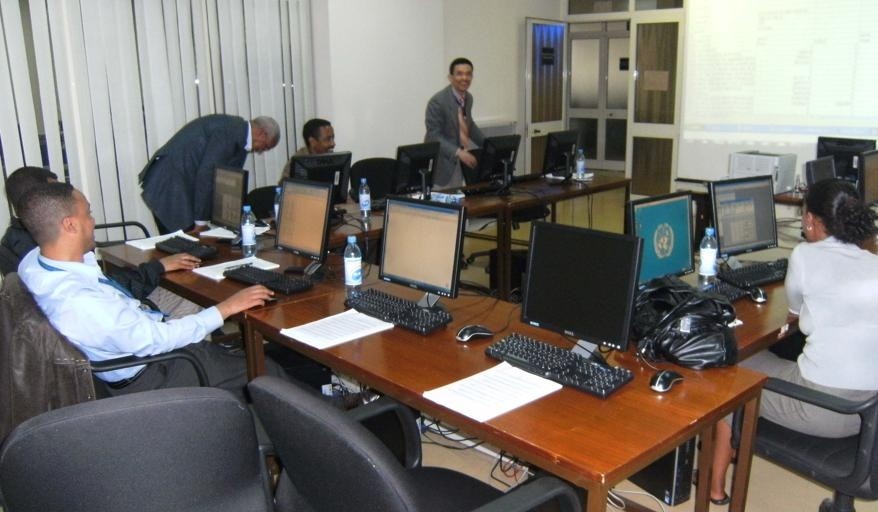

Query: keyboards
[705,281,746,304]
[371,197,386,212]
[223,263,314,295]
[717,257,788,289]
[344,288,452,336]
[464,187,501,196]
[155,236,217,262]
[485,332,633,400]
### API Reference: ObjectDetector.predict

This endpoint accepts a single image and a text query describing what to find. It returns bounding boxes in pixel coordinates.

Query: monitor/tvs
[857,149,878,206]
[520,221,644,358]
[209,165,249,230]
[478,134,521,189]
[273,177,334,276]
[542,130,581,180]
[805,154,836,185]
[290,151,352,216]
[708,175,778,269]
[394,141,440,200]
[817,137,876,183]
[378,196,467,307]
[624,190,696,290]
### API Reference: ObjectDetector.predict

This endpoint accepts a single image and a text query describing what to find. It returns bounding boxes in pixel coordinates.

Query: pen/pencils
[226,263,253,269]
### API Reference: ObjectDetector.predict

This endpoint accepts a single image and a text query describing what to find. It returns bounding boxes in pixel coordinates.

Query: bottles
[697,227,718,283]
[240,206,256,258]
[343,236,363,296]
[359,177,371,220]
[274,187,281,223]
[576,147,585,178]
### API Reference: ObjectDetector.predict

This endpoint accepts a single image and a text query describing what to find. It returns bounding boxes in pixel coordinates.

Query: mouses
[649,370,684,392]
[750,286,767,304]
[456,324,494,342]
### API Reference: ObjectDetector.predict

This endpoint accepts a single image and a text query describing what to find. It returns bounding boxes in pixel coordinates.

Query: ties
[457,95,468,150]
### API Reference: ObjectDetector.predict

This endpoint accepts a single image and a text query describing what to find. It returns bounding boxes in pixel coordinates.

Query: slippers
[692,440,736,505]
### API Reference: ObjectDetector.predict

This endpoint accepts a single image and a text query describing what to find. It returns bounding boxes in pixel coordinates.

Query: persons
[0,165,204,302]
[689,176,877,506]
[422,56,488,270]
[135,113,281,237]
[276,116,336,186]
[14,180,297,404]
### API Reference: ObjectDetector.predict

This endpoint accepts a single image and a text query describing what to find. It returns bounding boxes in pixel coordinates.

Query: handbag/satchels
[631,275,736,370]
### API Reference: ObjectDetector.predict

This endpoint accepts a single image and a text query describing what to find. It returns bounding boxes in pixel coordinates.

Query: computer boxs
[627,437,696,507]
